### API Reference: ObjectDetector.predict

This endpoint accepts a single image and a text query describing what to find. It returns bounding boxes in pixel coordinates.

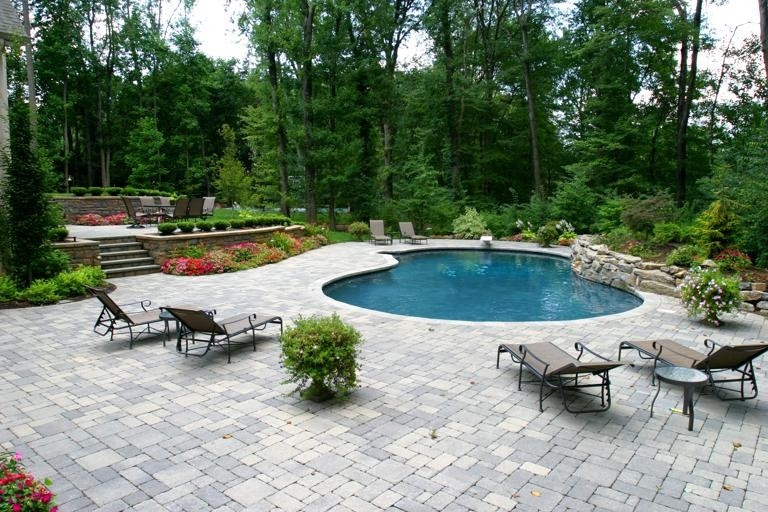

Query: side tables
[650,366,709,431]
[387,232,401,244]
[160,312,180,347]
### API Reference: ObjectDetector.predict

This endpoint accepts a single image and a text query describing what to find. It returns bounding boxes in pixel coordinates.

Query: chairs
[619,339,768,401]
[81,284,170,349]
[123,196,216,228]
[497,341,624,413]
[159,306,282,364]
[369,219,393,246]
[399,221,429,245]
